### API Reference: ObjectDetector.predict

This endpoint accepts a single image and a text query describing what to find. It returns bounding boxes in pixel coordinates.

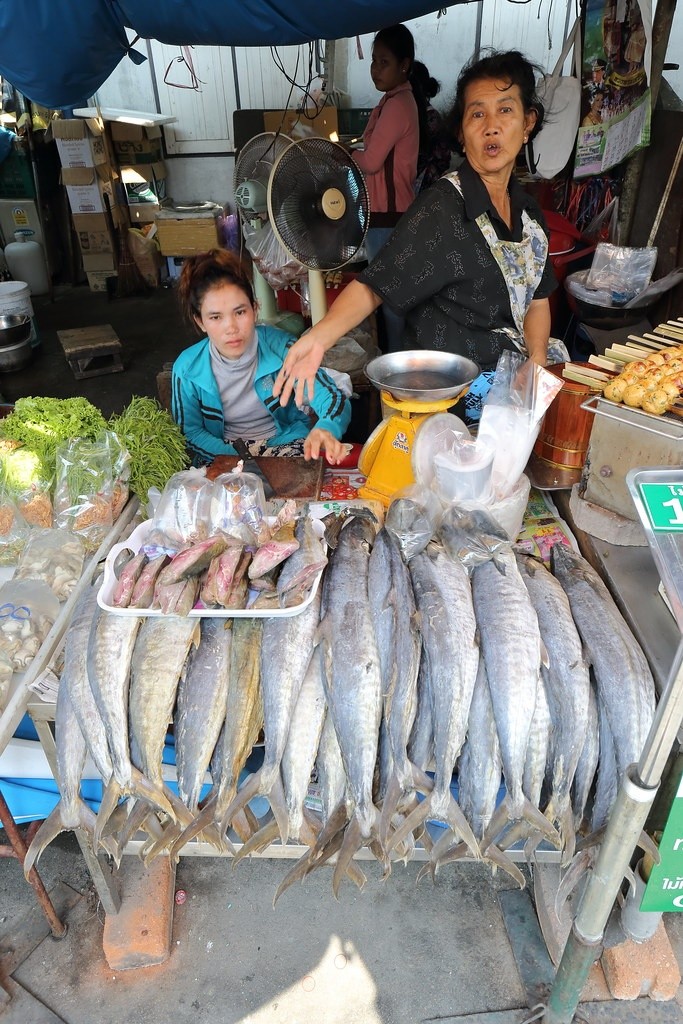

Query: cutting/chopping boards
[207,454,324,503]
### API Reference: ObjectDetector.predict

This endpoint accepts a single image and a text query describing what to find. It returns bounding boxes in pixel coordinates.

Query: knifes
[233,437,276,503]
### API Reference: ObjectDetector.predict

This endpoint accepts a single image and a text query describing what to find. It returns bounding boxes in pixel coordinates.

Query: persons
[174,248,353,467]
[582,91,604,127]
[339,22,452,264]
[583,59,611,109]
[271,48,561,411]
[580,127,604,143]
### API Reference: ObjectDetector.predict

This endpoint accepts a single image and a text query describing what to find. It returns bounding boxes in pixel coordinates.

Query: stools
[57,323,124,380]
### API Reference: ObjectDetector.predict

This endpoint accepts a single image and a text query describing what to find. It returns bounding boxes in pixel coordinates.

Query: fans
[267,136,371,326]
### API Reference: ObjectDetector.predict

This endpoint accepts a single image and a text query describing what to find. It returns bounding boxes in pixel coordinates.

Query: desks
[27,692,606,915]
[232,131,310,337]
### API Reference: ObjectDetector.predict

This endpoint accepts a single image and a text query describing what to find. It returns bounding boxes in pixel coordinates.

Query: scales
[357,349,482,510]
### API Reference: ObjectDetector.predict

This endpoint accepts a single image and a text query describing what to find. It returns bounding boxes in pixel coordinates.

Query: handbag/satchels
[524,16,582,180]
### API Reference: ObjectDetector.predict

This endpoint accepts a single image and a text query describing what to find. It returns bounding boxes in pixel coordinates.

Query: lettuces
[0,396,106,493]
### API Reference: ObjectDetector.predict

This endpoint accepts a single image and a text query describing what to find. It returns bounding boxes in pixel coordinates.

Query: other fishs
[20,502,661,924]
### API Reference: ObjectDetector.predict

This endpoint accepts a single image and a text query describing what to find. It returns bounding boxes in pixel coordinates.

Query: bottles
[4,232,50,296]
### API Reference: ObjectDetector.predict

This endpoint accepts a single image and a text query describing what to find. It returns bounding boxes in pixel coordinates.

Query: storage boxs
[42,118,229,292]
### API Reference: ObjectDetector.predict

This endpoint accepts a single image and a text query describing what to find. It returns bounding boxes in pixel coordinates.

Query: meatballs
[604,346,683,414]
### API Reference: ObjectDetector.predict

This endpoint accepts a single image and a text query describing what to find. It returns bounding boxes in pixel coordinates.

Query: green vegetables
[67,395,192,527]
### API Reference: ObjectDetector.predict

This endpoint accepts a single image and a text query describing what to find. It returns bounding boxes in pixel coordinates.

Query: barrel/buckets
[549,232,594,332]
[0,281,41,348]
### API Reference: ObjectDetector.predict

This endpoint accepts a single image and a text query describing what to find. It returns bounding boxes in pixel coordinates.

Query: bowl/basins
[0,337,31,371]
[564,281,661,331]
[0,314,31,346]
